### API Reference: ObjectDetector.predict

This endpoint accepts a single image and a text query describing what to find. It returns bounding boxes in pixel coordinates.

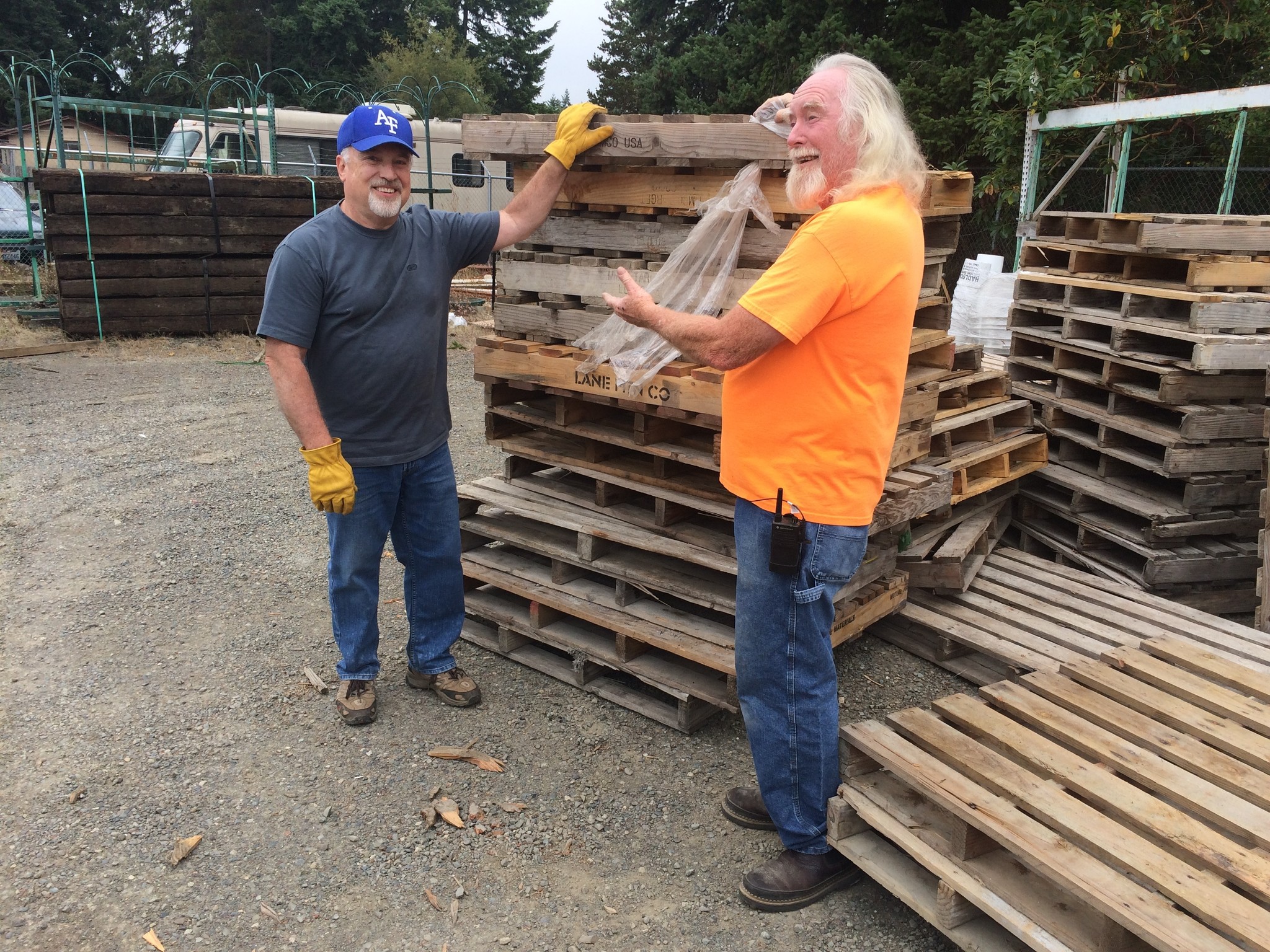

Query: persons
[600,55,928,913]
[256,99,615,728]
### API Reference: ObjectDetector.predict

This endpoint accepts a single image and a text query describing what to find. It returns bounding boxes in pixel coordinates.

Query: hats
[335,104,420,159]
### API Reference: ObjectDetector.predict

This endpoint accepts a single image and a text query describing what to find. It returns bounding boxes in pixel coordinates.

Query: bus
[145,102,514,213]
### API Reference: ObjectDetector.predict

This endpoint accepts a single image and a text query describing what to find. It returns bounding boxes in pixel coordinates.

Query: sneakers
[405,665,481,708]
[335,681,377,726]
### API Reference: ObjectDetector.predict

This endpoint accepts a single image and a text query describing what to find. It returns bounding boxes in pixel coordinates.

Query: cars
[0,181,45,265]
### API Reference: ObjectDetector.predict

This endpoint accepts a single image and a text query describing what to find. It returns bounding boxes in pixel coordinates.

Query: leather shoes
[722,786,777,831]
[740,850,861,912]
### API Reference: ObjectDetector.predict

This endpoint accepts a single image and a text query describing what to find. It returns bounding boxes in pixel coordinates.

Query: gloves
[543,103,614,170]
[300,437,358,515]
